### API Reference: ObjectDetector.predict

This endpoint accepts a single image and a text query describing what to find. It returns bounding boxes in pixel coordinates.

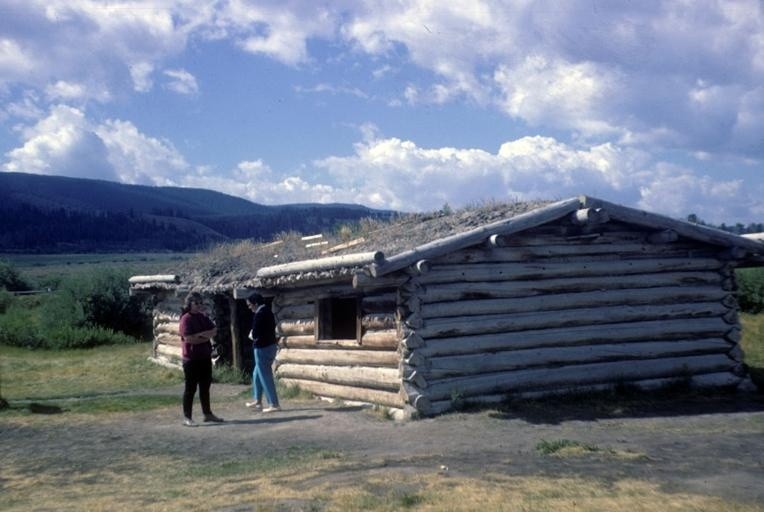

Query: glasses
[192,302,203,306]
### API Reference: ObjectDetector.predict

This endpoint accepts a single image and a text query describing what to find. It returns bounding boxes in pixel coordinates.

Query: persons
[178,291,225,427]
[244,291,283,414]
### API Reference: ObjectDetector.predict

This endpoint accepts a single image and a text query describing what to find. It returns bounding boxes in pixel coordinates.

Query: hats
[183,290,203,305]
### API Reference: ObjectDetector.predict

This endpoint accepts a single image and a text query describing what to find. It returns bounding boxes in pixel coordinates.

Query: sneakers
[201,413,225,424]
[181,416,200,428]
[261,405,282,413]
[244,400,264,409]
